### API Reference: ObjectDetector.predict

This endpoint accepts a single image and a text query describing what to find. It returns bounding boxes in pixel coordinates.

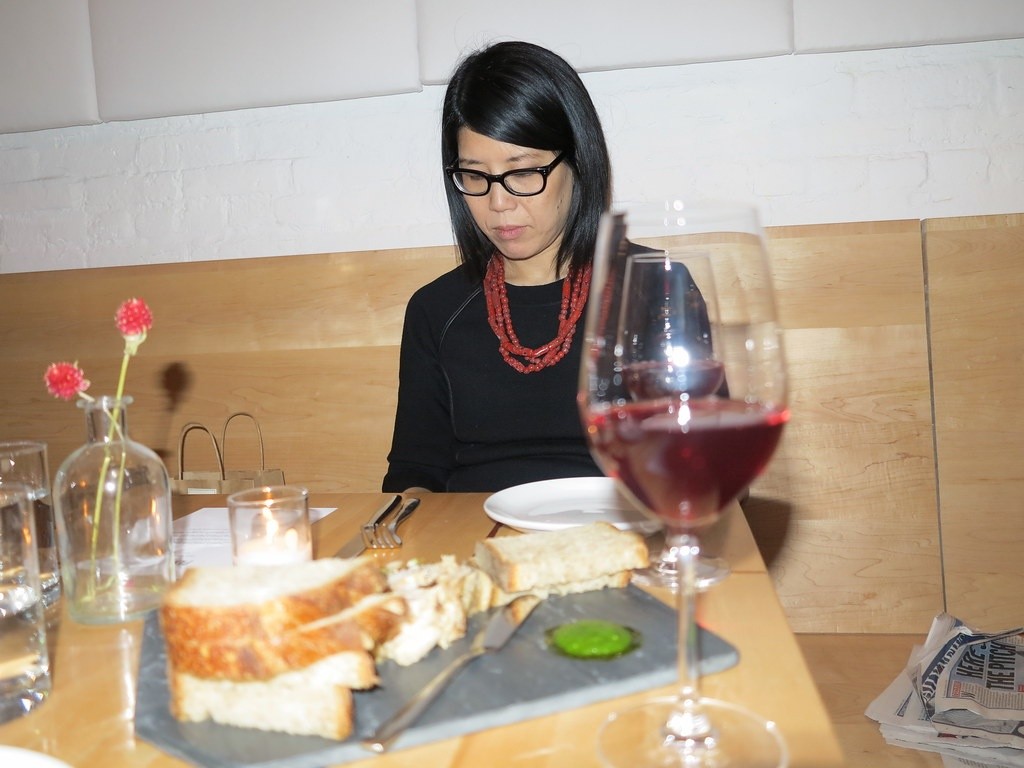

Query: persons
[381,41,711,494]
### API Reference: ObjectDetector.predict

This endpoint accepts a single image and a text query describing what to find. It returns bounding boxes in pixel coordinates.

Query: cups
[0,438,64,606]
[0,451,53,725]
[226,482,313,566]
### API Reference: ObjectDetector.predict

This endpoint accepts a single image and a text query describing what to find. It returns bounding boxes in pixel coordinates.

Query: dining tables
[1,476,845,767]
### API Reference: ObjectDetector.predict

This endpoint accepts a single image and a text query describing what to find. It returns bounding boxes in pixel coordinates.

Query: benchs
[0,214,1024,768]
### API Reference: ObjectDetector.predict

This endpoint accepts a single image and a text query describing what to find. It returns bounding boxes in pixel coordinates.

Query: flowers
[44,298,155,604]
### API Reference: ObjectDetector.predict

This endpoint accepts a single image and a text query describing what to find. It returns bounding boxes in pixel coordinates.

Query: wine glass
[618,249,739,587]
[576,202,790,768]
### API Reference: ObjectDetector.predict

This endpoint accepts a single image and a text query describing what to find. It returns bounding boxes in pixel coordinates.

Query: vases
[52,395,176,627]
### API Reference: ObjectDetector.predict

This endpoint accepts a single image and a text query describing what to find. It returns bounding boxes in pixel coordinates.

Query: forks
[362,498,423,551]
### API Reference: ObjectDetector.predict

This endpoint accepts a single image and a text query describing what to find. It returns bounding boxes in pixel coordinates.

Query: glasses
[446,146,571,197]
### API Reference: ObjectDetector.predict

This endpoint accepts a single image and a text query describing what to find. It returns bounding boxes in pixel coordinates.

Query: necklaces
[483,250,594,374]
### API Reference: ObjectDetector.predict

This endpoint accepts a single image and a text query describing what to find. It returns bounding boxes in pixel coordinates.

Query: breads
[472,520,652,609]
[156,552,412,740]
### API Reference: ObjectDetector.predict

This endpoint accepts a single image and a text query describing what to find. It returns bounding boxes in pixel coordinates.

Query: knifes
[363,591,544,757]
[330,495,405,570]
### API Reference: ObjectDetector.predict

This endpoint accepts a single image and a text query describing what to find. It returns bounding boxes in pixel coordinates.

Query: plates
[483,477,673,534]
[136,579,742,768]
